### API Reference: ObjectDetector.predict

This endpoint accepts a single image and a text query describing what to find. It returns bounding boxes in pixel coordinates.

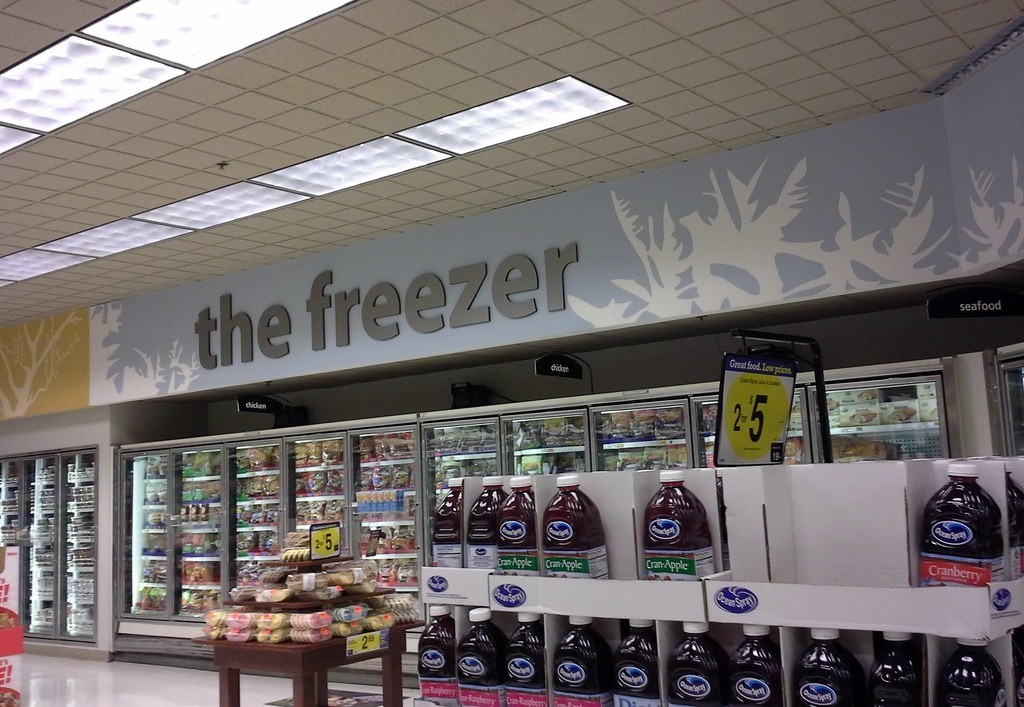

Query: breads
[862,391,876,399]
[890,409,916,420]
[852,412,877,422]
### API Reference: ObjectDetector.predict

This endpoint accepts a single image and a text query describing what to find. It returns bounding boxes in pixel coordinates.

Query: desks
[191,620,426,707]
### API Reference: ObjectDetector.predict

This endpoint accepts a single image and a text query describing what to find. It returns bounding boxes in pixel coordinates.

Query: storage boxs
[704,456,1024,642]
[0,546,20,630]
[0,625,24,707]
[420,476,533,607]
[488,468,724,623]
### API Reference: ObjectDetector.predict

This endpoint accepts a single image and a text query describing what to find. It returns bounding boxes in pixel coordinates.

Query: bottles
[728,625,783,707]
[543,475,609,579]
[643,472,716,581]
[1006,461,1024,581]
[418,605,458,707]
[935,638,1008,707]
[667,622,728,707]
[458,608,506,707]
[613,619,662,707]
[466,476,538,570]
[792,628,867,707]
[919,462,1005,588]
[868,631,923,707]
[553,616,613,707]
[433,479,463,567]
[506,613,548,707]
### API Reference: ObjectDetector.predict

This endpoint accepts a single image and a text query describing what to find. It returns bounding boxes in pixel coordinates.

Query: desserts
[204,533,419,642]
